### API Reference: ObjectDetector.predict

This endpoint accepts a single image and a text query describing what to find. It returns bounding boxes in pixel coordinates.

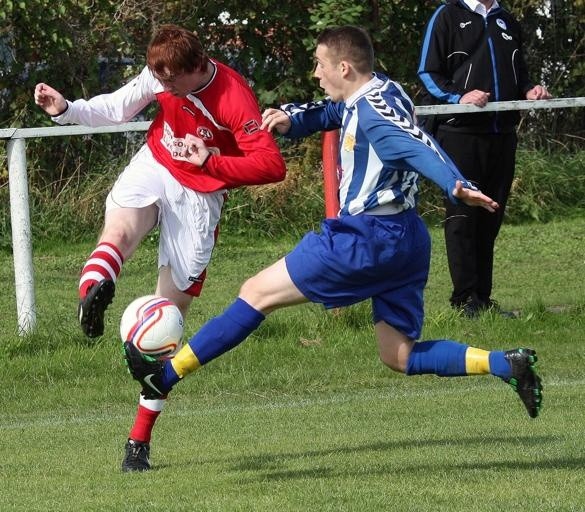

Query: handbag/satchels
[411,84,443,137]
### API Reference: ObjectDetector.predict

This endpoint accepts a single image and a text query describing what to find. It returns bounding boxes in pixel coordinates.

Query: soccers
[120,295,184,358]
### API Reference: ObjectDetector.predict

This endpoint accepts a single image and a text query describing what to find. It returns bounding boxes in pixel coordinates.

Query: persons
[122,25,541,412]
[32,23,284,471]
[418,0,552,319]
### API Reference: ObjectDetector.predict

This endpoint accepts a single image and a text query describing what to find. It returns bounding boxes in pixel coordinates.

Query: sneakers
[123,341,173,400]
[504,348,543,419]
[122,441,151,472]
[78,279,115,338]
